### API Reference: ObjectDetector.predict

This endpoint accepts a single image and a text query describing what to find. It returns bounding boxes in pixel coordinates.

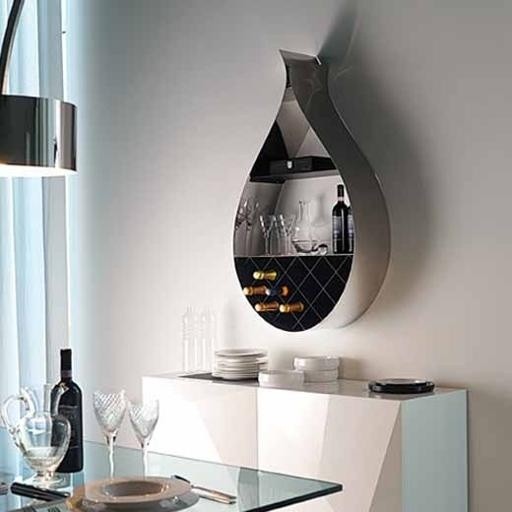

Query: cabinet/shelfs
[229,48,391,332]
[143,369,469,512]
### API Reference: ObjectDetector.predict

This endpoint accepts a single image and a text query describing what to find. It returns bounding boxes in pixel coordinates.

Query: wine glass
[257,212,293,257]
[91,387,126,480]
[127,398,160,478]
[235,196,261,256]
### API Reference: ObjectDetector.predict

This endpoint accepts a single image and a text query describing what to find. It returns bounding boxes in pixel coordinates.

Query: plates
[367,376,436,394]
[211,346,267,380]
[73,474,192,506]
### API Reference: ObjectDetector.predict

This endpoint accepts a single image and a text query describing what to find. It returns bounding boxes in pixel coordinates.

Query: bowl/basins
[259,369,305,388]
[293,356,339,371]
[1,94,76,167]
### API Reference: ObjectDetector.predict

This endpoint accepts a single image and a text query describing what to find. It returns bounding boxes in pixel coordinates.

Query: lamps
[0,0,79,179]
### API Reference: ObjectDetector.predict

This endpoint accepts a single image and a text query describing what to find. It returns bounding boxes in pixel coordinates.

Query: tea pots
[0,383,72,485]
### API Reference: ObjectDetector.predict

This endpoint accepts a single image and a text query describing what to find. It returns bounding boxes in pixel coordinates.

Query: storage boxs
[268,155,338,174]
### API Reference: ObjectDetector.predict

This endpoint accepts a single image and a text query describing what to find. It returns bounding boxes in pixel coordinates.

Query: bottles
[181,301,214,374]
[290,200,321,254]
[50,348,84,472]
[319,244,328,253]
[331,183,354,254]
